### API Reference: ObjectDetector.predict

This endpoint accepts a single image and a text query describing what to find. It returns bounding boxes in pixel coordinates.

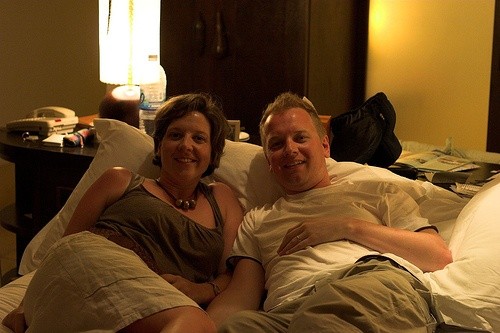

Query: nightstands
[0,134,96,276]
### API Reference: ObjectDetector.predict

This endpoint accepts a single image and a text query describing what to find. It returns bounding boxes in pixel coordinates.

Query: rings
[297,236,302,243]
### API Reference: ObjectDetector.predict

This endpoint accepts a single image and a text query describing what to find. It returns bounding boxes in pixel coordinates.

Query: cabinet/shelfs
[161,0,371,146]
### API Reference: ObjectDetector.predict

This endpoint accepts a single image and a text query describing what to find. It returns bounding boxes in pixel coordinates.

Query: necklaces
[153,176,198,210]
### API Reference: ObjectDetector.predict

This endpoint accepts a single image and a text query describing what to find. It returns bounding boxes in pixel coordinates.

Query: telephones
[6,106,78,137]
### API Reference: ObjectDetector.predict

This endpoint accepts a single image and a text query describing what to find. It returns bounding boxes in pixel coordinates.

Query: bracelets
[208,281,221,297]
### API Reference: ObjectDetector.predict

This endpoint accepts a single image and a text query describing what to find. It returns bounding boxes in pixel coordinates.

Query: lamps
[99,0,161,131]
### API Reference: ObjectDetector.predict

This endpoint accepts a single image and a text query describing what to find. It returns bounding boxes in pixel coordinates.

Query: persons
[206,93,453,333]
[4,94,244,333]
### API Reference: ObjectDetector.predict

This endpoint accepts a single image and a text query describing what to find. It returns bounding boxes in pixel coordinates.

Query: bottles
[139,54,167,137]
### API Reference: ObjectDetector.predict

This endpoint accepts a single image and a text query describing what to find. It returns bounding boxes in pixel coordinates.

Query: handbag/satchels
[330,91,402,169]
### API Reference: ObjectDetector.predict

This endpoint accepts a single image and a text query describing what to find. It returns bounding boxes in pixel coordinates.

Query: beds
[0,116,500,333]
